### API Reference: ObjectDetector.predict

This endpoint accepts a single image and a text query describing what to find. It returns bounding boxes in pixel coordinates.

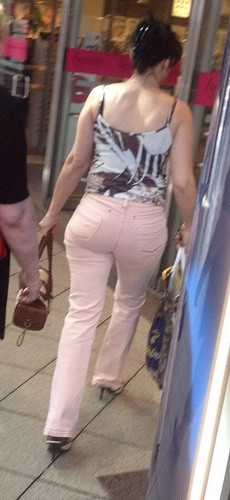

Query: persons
[38,18,198,461]
[0,81,42,340]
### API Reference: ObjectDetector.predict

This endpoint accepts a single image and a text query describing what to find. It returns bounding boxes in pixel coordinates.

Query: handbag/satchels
[145,240,188,388]
[14,218,60,345]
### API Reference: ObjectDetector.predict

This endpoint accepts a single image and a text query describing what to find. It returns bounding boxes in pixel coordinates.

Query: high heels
[94,380,127,401]
[44,438,76,462]
